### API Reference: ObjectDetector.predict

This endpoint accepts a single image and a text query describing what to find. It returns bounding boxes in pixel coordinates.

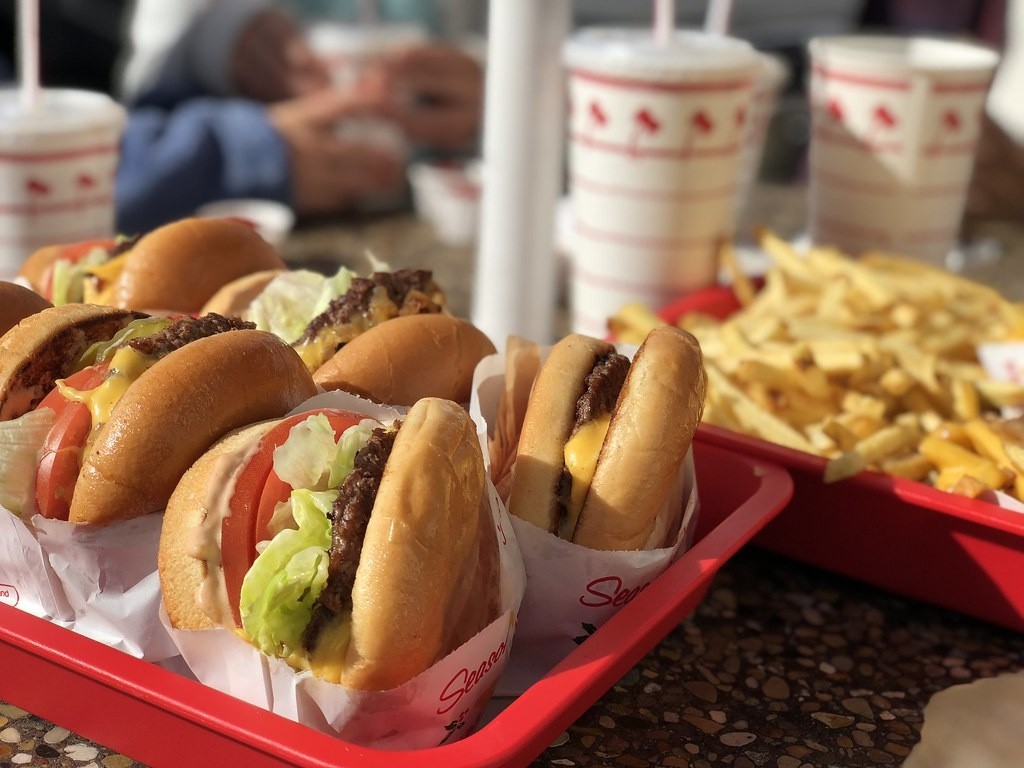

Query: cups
[809,34,1000,272]
[0,86,129,282]
[563,25,786,338]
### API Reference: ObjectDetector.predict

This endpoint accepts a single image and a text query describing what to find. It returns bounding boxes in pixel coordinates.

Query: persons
[0,0,402,247]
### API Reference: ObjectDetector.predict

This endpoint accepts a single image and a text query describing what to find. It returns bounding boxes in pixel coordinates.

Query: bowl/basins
[0,440,790,768]
[596,272,1024,633]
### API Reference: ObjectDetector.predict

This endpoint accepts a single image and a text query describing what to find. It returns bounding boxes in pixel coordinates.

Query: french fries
[609,227,1024,503]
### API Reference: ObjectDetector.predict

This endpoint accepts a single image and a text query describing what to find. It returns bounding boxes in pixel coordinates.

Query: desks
[0,186,1024,768]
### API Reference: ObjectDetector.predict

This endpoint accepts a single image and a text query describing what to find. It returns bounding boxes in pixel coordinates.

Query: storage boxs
[599,277,1024,623]
[0,438,792,768]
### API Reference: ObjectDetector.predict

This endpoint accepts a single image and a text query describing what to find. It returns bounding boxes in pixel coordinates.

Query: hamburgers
[0,215,707,691]
